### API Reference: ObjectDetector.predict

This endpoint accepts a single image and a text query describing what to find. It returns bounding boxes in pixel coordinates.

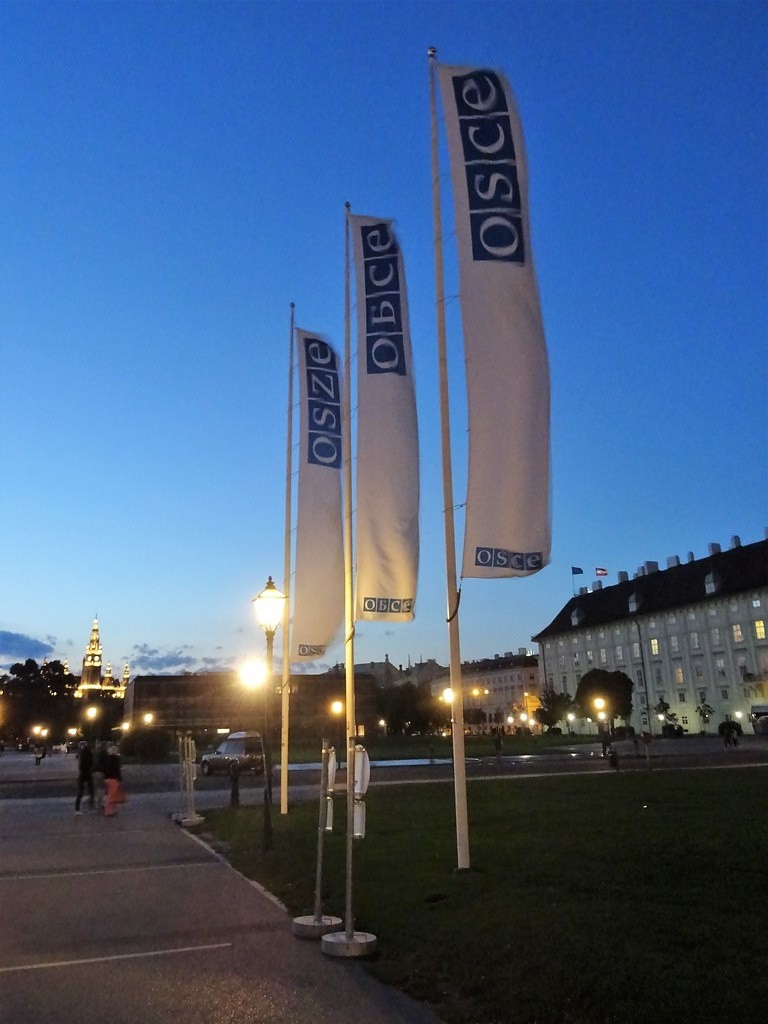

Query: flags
[572,567,583,574]
[596,568,607,576]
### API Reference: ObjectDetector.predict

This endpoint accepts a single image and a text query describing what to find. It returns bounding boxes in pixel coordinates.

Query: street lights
[249,574,287,847]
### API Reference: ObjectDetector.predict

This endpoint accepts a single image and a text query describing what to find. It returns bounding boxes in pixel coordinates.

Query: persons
[0,740,5,757]
[103,746,122,817]
[494,733,503,759]
[75,746,95,815]
[724,725,741,750]
[34,744,42,765]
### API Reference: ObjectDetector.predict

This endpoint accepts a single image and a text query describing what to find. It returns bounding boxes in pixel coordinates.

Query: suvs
[201,731,269,779]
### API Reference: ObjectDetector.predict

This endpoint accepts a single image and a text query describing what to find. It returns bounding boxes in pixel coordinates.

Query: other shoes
[75,811,82,815]
[105,811,118,818]
